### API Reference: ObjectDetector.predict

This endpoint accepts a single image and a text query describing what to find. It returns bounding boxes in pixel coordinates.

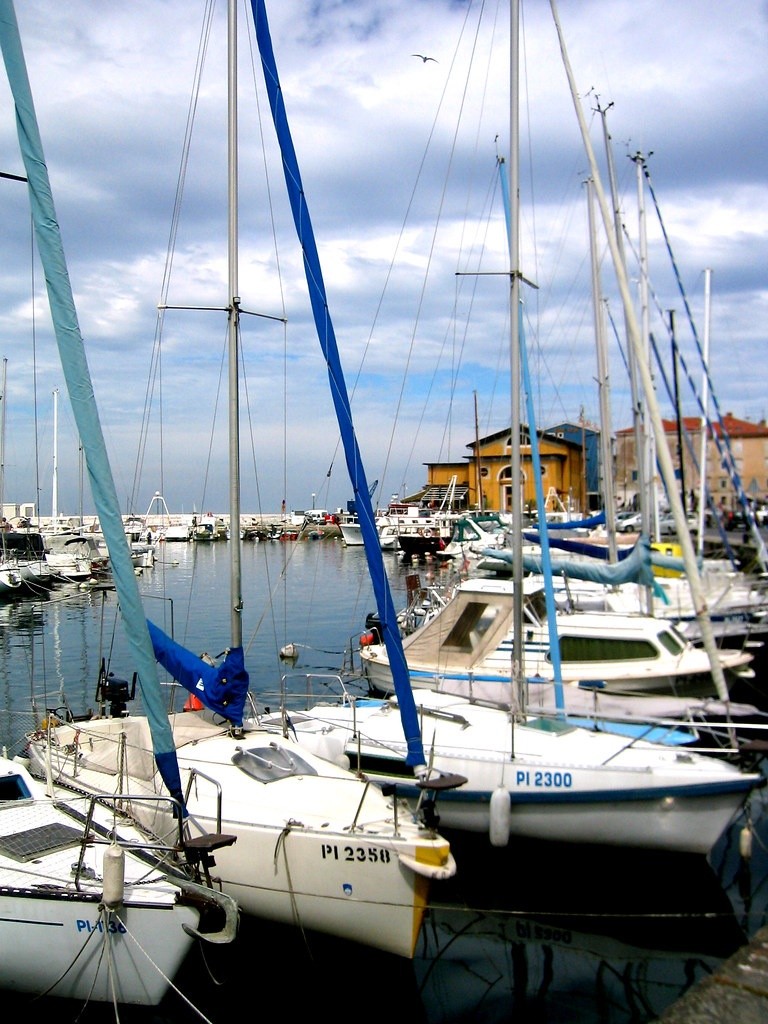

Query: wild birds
[409,51,440,65]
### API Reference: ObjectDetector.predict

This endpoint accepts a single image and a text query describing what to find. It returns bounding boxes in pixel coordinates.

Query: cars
[724,509,756,532]
[661,510,700,535]
[615,510,663,534]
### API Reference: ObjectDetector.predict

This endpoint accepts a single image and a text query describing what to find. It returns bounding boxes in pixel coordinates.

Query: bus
[303,509,329,524]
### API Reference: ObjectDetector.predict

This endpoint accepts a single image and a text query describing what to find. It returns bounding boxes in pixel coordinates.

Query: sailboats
[0,345,337,598]
[242,0,768,858]
[23,0,458,959]
[336,89,767,739]
[0,0,240,1008]
